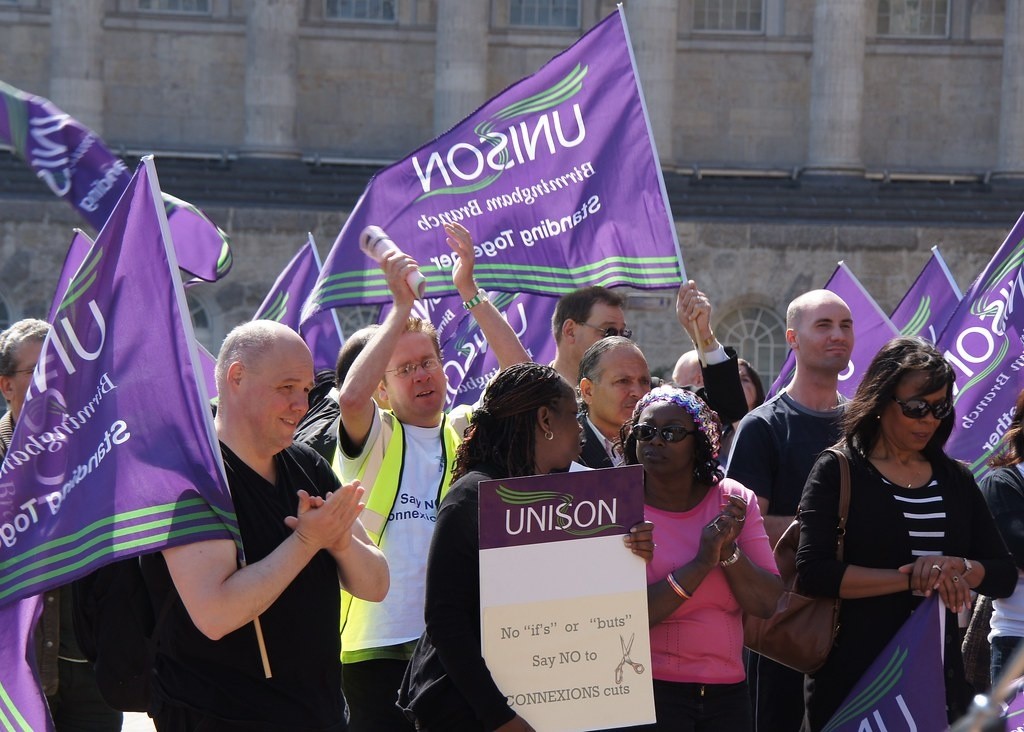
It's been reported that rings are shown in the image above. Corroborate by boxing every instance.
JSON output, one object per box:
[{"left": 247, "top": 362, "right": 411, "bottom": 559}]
[
  {"left": 651, "top": 543, "right": 657, "bottom": 552},
  {"left": 735, "top": 515, "right": 745, "bottom": 522},
  {"left": 714, "top": 523, "right": 721, "bottom": 532},
  {"left": 952, "top": 576, "right": 958, "bottom": 582},
  {"left": 931, "top": 565, "right": 942, "bottom": 571}
]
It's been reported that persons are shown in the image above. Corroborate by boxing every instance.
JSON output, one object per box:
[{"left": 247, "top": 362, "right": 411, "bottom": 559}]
[
  {"left": 551, "top": 277, "right": 857, "bottom": 732},
  {"left": 610, "top": 387, "right": 782, "bottom": 732},
  {"left": 794, "top": 339, "right": 1024, "bottom": 732},
  {"left": 0, "top": 320, "right": 74, "bottom": 732},
  {"left": 397, "top": 361, "right": 655, "bottom": 732},
  {"left": 143, "top": 321, "right": 390, "bottom": 732},
  {"left": 330, "top": 223, "right": 533, "bottom": 732}
]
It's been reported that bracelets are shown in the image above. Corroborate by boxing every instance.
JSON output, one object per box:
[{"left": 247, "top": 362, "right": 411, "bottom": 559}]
[
  {"left": 720, "top": 545, "right": 740, "bottom": 567},
  {"left": 666, "top": 571, "right": 692, "bottom": 600},
  {"left": 692, "top": 330, "right": 715, "bottom": 349}
]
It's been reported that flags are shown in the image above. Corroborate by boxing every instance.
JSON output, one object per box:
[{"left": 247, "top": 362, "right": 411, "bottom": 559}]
[
  {"left": 252, "top": 9, "right": 683, "bottom": 413},
  {"left": 765, "top": 212, "right": 1024, "bottom": 484},
  {"left": 821, "top": 591, "right": 947, "bottom": 732},
  {"left": 0, "top": 84, "right": 244, "bottom": 732}
]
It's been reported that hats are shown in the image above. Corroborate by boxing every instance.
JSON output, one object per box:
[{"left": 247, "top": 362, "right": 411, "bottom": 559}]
[{"left": 633, "top": 385, "right": 721, "bottom": 459}]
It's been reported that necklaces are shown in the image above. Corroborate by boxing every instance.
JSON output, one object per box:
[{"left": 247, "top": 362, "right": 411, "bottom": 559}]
[{"left": 878, "top": 455, "right": 923, "bottom": 487}]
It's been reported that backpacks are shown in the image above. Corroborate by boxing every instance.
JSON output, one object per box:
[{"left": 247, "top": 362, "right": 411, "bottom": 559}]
[{"left": 72, "top": 558, "right": 178, "bottom": 712}]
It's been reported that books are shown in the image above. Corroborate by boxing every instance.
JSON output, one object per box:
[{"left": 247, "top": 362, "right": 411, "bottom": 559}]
[{"left": 359, "top": 226, "right": 426, "bottom": 301}]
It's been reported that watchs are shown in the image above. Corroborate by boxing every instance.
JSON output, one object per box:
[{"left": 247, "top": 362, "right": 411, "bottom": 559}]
[
  {"left": 961, "top": 558, "right": 971, "bottom": 579},
  {"left": 463, "top": 288, "right": 488, "bottom": 309}
]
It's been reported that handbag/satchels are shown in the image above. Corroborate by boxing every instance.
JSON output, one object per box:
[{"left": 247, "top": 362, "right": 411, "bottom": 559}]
[{"left": 742, "top": 448, "right": 851, "bottom": 672}]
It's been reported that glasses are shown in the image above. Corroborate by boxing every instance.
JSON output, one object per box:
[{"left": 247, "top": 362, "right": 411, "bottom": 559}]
[
  {"left": 633, "top": 425, "right": 696, "bottom": 443},
  {"left": 578, "top": 321, "right": 632, "bottom": 338},
  {"left": 892, "top": 397, "right": 953, "bottom": 419},
  {"left": 385, "top": 357, "right": 444, "bottom": 378}
]
